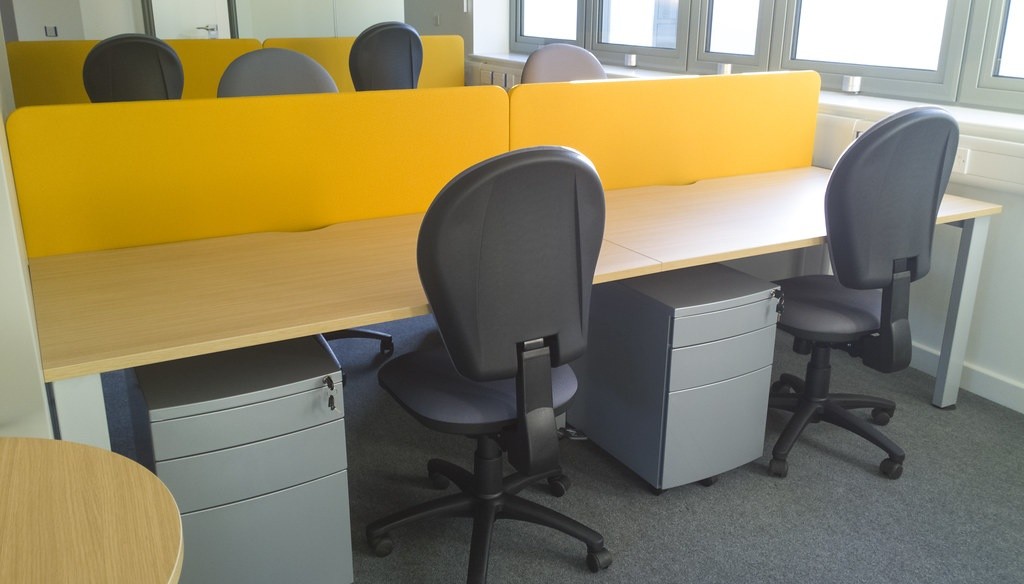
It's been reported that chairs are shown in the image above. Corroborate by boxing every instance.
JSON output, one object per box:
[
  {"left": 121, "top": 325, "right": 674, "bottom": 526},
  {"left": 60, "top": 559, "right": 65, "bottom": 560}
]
[
  {"left": 347, "top": 22, "right": 423, "bottom": 92},
  {"left": 769, "top": 106, "right": 960, "bottom": 481},
  {"left": 216, "top": 46, "right": 394, "bottom": 382},
  {"left": 82, "top": 33, "right": 184, "bottom": 104},
  {"left": 521, "top": 43, "right": 608, "bottom": 84},
  {"left": 365, "top": 146, "right": 613, "bottom": 584}
]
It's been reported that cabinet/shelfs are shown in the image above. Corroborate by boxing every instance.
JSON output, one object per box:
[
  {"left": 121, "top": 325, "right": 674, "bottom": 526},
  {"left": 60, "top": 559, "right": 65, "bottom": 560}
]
[
  {"left": 134, "top": 332, "right": 353, "bottom": 584},
  {"left": 565, "top": 261, "right": 781, "bottom": 496}
]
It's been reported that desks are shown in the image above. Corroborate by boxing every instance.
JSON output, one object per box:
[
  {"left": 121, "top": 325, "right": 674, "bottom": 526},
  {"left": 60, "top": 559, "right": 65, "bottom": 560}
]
[
  {"left": 0, "top": 435, "right": 183, "bottom": 584},
  {"left": 30, "top": 164, "right": 1005, "bottom": 451}
]
